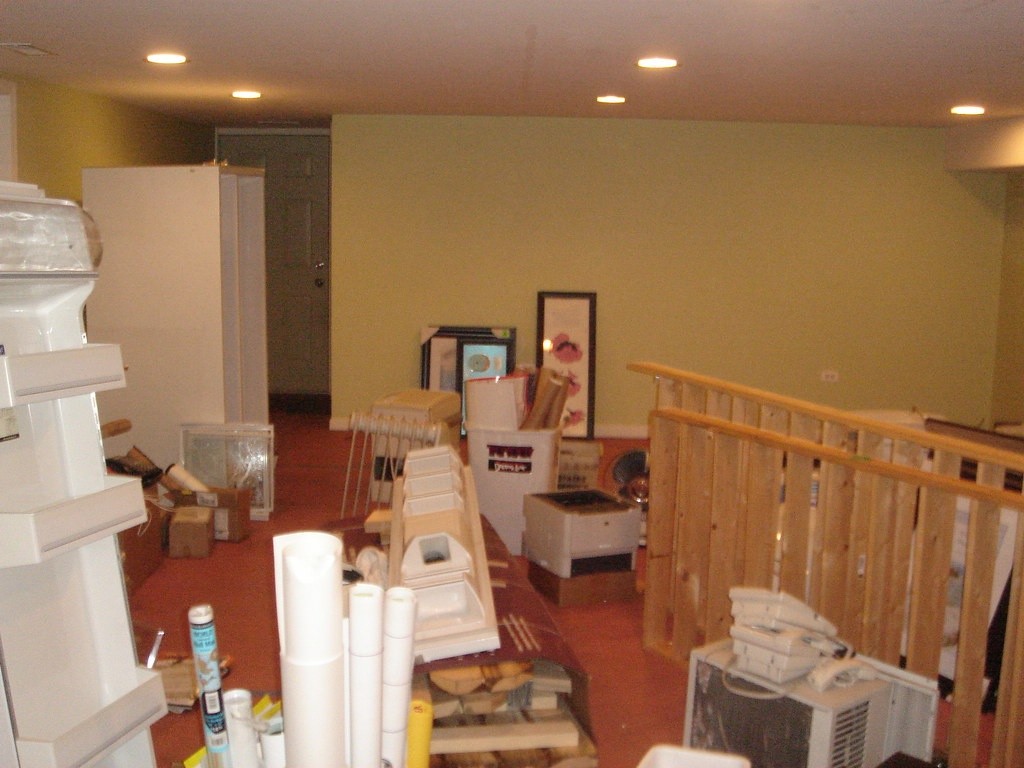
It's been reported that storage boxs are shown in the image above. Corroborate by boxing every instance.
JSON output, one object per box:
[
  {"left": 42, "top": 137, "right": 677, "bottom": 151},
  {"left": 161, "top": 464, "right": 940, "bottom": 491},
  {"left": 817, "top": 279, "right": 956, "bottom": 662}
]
[
  {"left": 389, "top": 514, "right": 595, "bottom": 768},
  {"left": 115, "top": 483, "right": 253, "bottom": 595}
]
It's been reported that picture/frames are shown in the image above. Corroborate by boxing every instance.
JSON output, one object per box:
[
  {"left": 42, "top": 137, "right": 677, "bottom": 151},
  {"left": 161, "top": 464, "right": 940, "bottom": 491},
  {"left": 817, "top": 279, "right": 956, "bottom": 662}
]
[
  {"left": 420, "top": 326, "right": 517, "bottom": 440},
  {"left": 536, "top": 290, "right": 595, "bottom": 442}
]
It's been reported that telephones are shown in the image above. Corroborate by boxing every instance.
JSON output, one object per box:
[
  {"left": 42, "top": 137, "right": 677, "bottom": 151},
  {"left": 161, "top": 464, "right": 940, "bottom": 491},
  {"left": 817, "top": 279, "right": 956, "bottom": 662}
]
[
  {"left": 729, "top": 586, "right": 840, "bottom": 636},
  {"left": 729, "top": 625, "right": 877, "bottom": 694}
]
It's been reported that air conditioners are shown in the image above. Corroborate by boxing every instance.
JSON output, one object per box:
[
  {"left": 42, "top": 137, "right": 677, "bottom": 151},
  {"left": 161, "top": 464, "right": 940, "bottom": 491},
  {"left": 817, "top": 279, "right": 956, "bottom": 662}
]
[{"left": 683, "top": 638, "right": 893, "bottom": 768}]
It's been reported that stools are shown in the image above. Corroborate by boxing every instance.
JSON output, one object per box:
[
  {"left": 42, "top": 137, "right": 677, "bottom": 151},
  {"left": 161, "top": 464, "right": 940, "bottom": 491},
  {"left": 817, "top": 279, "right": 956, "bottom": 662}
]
[{"left": 518, "top": 487, "right": 643, "bottom": 608}]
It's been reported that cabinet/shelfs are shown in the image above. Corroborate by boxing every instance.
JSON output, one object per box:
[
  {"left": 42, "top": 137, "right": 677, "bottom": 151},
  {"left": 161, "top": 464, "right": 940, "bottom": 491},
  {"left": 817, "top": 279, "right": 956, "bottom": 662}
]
[
  {"left": 0, "top": 184, "right": 170, "bottom": 768},
  {"left": 79, "top": 163, "right": 266, "bottom": 478},
  {"left": 369, "top": 389, "right": 459, "bottom": 503}
]
